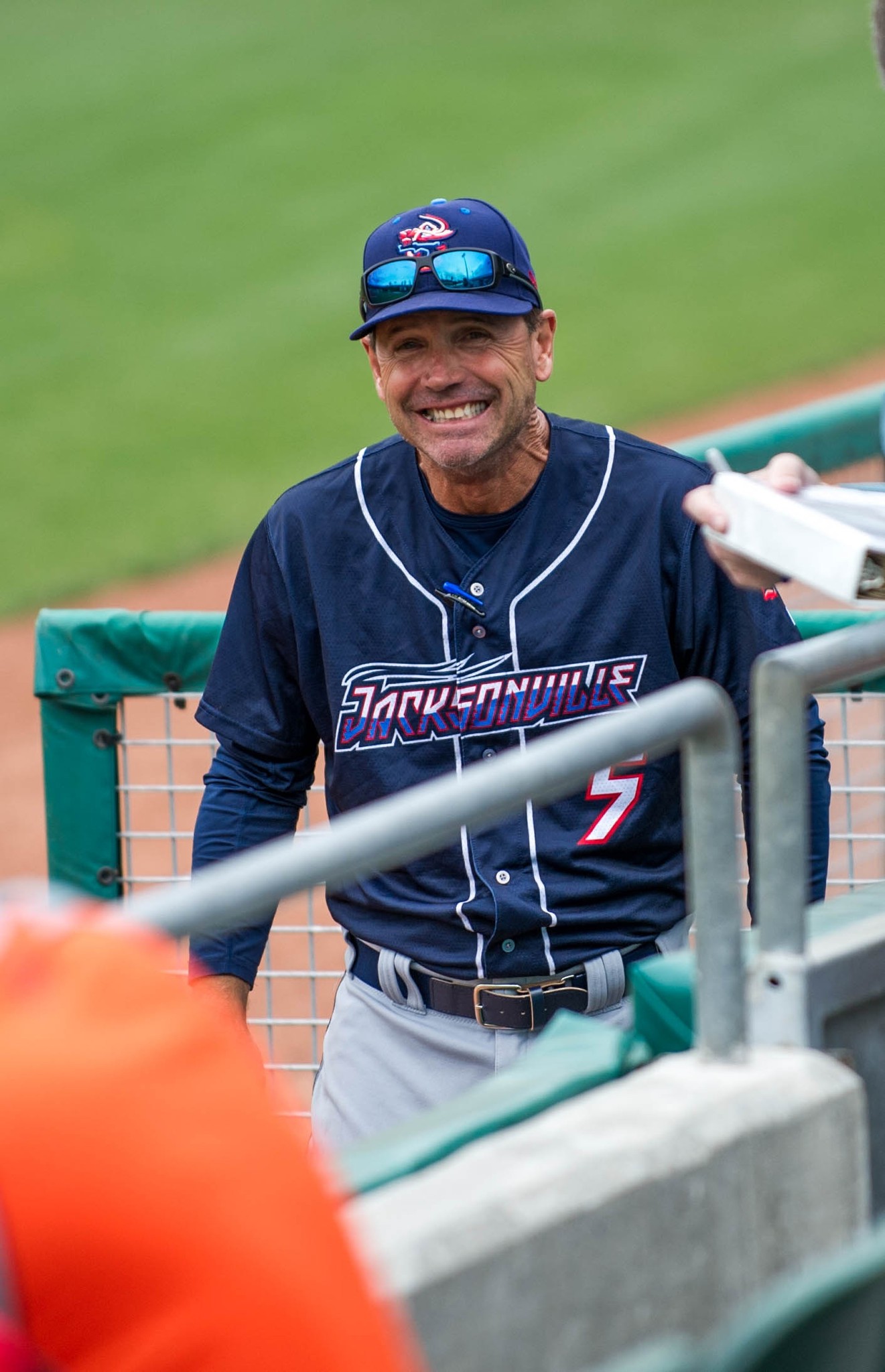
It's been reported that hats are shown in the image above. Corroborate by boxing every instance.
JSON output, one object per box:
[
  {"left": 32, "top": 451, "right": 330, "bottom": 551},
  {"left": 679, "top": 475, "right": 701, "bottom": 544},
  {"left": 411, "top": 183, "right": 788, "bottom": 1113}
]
[{"left": 349, "top": 196, "right": 541, "bottom": 342}]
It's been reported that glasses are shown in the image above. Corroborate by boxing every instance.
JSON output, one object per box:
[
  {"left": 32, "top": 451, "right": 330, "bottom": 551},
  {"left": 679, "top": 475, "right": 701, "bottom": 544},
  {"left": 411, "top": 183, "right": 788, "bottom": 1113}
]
[{"left": 359, "top": 248, "right": 543, "bottom": 323}]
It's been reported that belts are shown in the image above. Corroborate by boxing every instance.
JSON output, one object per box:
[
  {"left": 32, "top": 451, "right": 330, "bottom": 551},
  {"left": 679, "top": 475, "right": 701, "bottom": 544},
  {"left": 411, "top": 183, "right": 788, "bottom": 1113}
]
[{"left": 350, "top": 938, "right": 668, "bottom": 1034}]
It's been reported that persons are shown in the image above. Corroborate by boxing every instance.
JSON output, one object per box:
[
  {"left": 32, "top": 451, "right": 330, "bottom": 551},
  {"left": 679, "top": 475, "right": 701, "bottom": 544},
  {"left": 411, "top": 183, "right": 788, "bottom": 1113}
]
[
  {"left": 684, "top": 448, "right": 885, "bottom": 592},
  {"left": 186, "top": 188, "right": 831, "bottom": 1146}
]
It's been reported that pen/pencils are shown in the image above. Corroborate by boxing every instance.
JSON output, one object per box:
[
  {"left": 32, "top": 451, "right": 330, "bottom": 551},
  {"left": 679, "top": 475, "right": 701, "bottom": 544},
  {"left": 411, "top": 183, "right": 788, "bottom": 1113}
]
[{"left": 704, "top": 447, "right": 732, "bottom": 474}]
